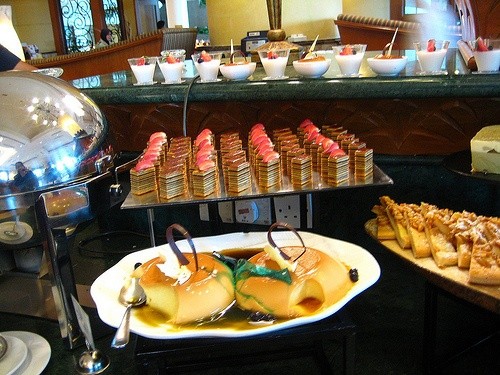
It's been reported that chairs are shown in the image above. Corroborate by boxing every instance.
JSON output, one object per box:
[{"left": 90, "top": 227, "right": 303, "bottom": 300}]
[{"left": 333, "top": 12, "right": 461, "bottom": 49}]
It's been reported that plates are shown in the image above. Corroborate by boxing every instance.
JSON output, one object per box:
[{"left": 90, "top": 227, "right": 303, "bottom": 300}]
[
  {"left": 30, "top": 67, "right": 63, "bottom": 78},
  {"left": 89, "top": 231, "right": 381, "bottom": 339},
  {"left": 0, "top": 331, "right": 52, "bottom": 375},
  {"left": 441, "top": 150, "right": 500, "bottom": 182},
  {"left": 0, "top": 221, "right": 34, "bottom": 245}
]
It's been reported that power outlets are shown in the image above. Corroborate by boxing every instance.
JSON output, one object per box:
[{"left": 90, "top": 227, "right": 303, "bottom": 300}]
[{"left": 273, "top": 194, "right": 312, "bottom": 229}]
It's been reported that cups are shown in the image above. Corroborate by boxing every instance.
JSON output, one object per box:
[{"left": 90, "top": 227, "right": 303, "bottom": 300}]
[
  {"left": 332, "top": 44, "right": 367, "bottom": 76},
  {"left": 128, "top": 57, "right": 157, "bottom": 83},
  {"left": 156, "top": 55, "right": 185, "bottom": 83},
  {"left": 413, "top": 41, "right": 450, "bottom": 72},
  {"left": 256, "top": 48, "right": 291, "bottom": 79},
  {"left": 191, "top": 50, "right": 223, "bottom": 81},
  {"left": 468, "top": 40, "right": 500, "bottom": 72}
]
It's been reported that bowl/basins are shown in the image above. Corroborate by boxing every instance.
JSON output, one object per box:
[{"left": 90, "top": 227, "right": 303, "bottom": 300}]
[
  {"left": 219, "top": 62, "right": 256, "bottom": 80},
  {"left": 367, "top": 55, "right": 409, "bottom": 77},
  {"left": 292, "top": 59, "right": 331, "bottom": 78}
]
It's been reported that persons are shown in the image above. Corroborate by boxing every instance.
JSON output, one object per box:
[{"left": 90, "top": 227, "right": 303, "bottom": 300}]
[
  {"left": 12, "top": 161, "right": 39, "bottom": 191},
  {"left": 42, "top": 162, "right": 59, "bottom": 183},
  {"left": 95, "top": 28, "right": 115, "bottom": 50}
]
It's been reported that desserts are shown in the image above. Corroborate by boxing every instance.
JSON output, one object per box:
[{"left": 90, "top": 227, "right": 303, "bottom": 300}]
[
  {"left": 125, "top": 222, "right": 353, "bottom": 325},
  {"left": 129, "top": 120, "right": 373, "bottom": 200}
]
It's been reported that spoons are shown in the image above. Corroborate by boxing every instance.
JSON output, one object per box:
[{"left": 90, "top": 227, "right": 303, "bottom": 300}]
[
  {"left": 0, "top": 335, "right": 8, "bottom": 360},
  {"left": 110, "top": 281, "right": 146, "bottom": 349},
  {"left": 3, "top": 215, "right": 20, "bottom": 237}
]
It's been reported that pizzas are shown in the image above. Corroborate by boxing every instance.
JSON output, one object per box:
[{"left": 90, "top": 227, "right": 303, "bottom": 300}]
[{"left": 371, "top": 194, "right": 500, "bottom": 287}]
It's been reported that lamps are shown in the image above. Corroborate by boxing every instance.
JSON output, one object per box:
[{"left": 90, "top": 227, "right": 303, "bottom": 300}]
[{"left": 0, "top": 10, "right": 27, "bottom": 62}]
